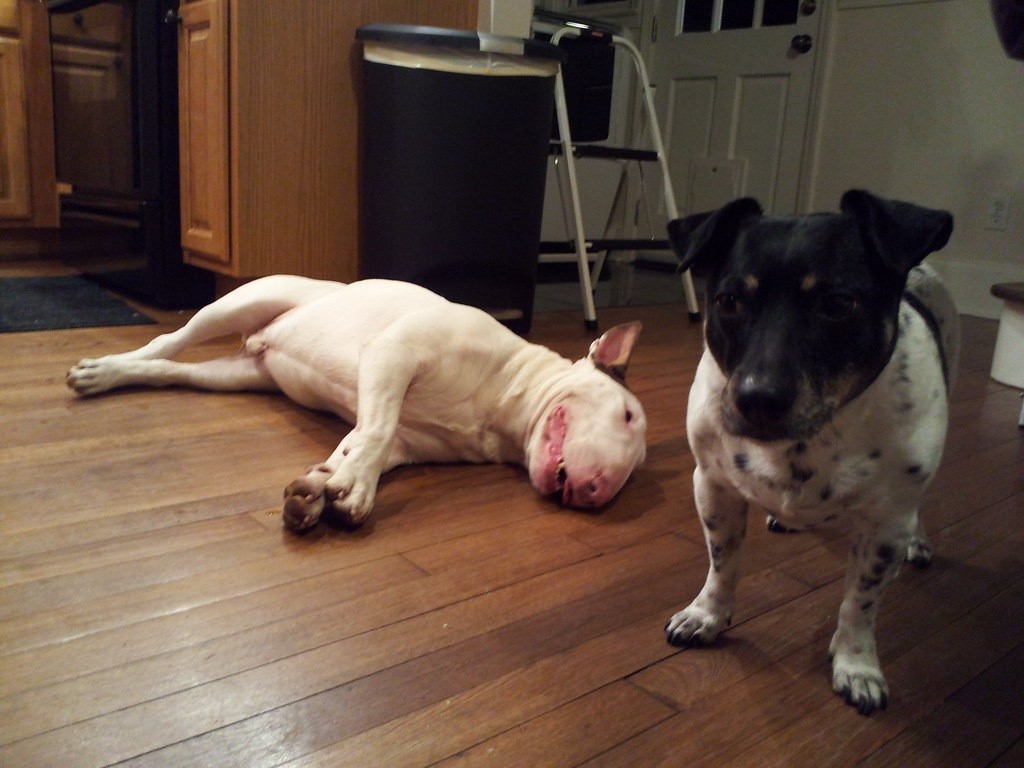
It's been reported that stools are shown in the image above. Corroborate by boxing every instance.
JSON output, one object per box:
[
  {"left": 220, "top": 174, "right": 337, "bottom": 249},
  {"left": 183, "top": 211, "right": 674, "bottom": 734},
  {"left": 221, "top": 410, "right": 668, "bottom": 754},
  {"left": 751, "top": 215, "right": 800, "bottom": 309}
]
[{"left": 988, "top": 281, "right": 1024, "bottom": 390}]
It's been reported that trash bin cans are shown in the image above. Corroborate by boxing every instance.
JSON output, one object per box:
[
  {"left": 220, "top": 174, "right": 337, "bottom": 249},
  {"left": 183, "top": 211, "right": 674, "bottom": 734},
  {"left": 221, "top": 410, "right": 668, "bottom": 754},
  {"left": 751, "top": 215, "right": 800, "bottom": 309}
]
[{"left": 355, "top": 23, "right": 565, "bottom": 334}]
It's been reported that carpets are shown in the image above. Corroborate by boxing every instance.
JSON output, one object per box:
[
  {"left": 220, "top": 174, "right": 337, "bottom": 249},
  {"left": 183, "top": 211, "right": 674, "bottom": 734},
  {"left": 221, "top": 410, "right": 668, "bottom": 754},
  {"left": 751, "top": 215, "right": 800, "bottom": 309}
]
[{"left": 0, "top": 274, "right": 160, "bottom": 333}]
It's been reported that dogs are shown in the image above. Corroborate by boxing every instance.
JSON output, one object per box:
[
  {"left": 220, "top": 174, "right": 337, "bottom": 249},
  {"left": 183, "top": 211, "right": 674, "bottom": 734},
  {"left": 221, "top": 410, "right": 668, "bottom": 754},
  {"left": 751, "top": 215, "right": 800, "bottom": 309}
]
[
  {"left": 663, "top": 186, "right": 964, "bottom": 720},
  {"left": 65, "top": 274, "right": 650, "bottom": 536}
]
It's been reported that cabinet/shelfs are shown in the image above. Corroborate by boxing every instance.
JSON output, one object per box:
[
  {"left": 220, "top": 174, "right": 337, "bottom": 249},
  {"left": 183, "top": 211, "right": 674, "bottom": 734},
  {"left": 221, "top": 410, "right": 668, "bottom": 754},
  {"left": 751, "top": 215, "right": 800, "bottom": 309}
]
[{"left": 0, "top": 0, "right": 480, "bottom": 301}]
